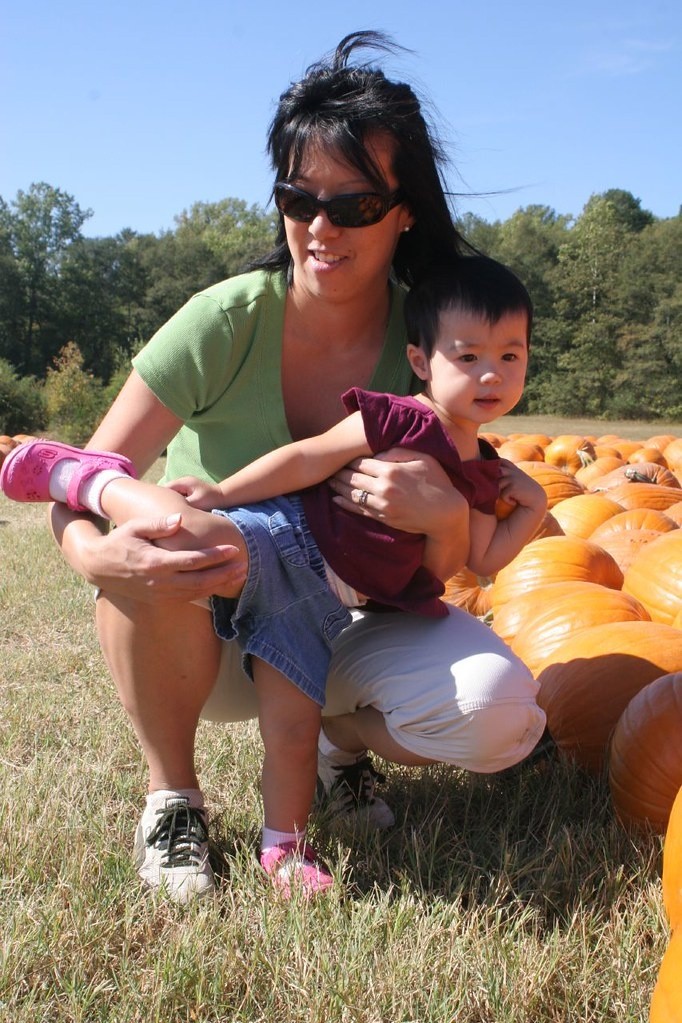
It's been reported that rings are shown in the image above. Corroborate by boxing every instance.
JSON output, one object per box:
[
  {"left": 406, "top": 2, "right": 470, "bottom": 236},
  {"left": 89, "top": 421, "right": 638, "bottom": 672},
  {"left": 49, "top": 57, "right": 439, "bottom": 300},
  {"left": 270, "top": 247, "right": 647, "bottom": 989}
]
[{"left": 358, "top": 490, "right": 374, "bottom": 505}]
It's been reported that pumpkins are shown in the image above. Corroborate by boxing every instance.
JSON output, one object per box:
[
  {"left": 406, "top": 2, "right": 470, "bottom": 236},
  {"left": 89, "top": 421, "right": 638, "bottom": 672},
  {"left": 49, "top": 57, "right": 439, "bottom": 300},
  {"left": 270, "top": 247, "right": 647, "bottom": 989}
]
[
  {"left": 442, "top": 432, "right": 682, "bottom": 1023},
  {"left": 0, "top": 434, "right": 34, "bottom": 471}
]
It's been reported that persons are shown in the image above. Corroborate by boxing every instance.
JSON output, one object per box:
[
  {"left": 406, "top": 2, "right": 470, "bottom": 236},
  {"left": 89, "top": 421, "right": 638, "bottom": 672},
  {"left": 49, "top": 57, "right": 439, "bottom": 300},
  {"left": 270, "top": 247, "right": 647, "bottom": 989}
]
[
  {"left": 1, "top": 257, "right": 547, "bottom": 908},
  {"left": 48, "top": 30, "right": 549, "bottom": 905}
]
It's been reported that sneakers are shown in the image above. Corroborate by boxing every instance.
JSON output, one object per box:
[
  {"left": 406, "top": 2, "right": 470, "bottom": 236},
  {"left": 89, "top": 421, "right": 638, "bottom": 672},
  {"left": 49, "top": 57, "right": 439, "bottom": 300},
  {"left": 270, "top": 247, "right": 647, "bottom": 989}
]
[
  {"left": 137, "top": 796, "right": 216, "bottom": 906},
  {"left": 317, "top": 749, "right": 395, "bottom": 834}
]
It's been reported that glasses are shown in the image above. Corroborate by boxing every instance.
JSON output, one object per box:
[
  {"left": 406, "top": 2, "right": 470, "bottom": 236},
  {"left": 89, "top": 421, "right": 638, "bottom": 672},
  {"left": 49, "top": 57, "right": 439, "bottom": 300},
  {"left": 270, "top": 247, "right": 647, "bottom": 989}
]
[{"left": 274, "top": 179, "right": 405, "bottom": 228}]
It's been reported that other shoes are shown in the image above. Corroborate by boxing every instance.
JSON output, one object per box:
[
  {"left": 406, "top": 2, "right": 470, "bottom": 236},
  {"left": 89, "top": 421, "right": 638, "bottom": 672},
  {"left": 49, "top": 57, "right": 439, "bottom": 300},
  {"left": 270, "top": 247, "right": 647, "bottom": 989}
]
[
  {"left": 260, "top": 841, "right": 347, "bottom": 909},
  {"left": 0, "top": 438, "right": 137, "bottom": 512}
]
